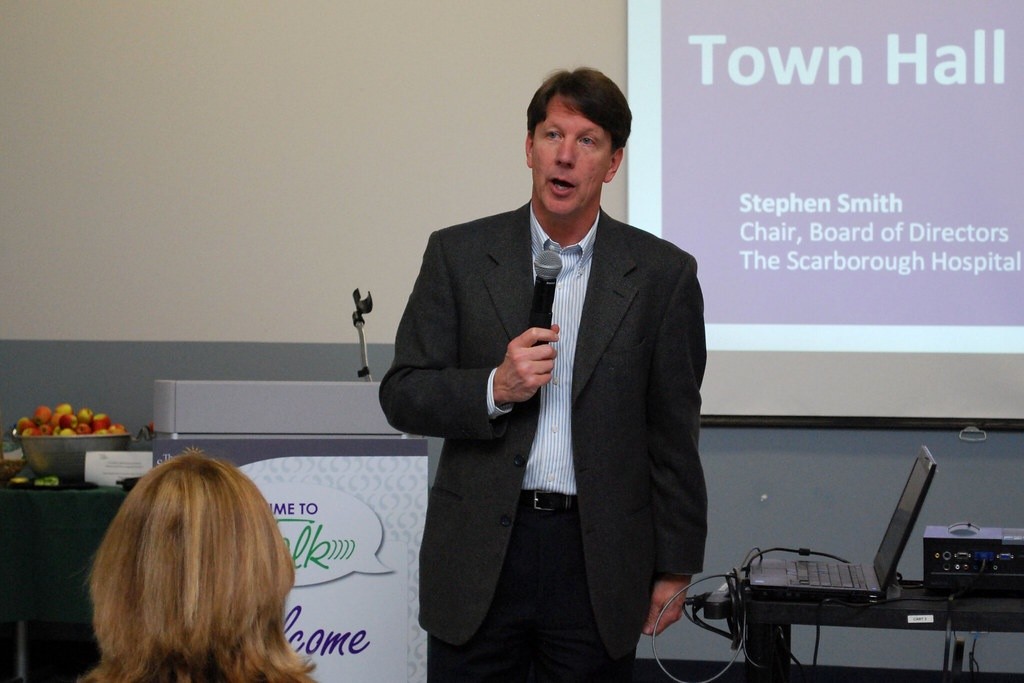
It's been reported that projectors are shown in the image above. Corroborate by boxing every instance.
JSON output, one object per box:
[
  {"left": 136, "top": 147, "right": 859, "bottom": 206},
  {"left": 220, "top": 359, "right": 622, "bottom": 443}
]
[{"left": 926, "top": 524, "right": 1024, "bottom": 599}]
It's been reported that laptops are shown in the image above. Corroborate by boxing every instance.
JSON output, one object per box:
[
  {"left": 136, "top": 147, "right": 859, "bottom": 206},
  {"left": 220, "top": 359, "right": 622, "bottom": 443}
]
[{"left": 749, "top": 445, "right": 937, "bottom": 601}]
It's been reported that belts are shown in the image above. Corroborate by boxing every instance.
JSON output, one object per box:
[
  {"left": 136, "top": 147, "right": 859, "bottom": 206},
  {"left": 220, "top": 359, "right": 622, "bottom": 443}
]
[{"left": 519, "top": 488, "right": 578, "bottom": 511}]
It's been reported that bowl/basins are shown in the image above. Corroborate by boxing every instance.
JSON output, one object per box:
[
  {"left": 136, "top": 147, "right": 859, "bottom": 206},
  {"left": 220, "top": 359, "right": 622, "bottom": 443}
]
[{"left": 15, "top": 434, "right": 133, "bottom": 480}]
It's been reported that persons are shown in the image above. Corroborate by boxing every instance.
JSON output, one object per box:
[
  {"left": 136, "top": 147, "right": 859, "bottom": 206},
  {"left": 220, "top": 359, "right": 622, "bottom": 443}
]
[
  {"left": 379, "top": 68, "right": 707, "bottom": 683},
  {"left": 79, "top": 453, "right": 318, "bottom": 683}
]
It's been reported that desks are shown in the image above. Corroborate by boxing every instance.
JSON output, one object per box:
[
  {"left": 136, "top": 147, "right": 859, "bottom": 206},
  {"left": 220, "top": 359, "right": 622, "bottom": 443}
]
[
  {"left": 702, "top": 572, "right": 1024, "bottom": 682},
  {"left": 0, "top": 484, "right": 127, "bottom": 682}
]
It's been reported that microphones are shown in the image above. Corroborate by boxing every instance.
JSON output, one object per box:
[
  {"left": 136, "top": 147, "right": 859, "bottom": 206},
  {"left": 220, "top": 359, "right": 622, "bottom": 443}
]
[{"left": 528, "top": 249, "right": 561, "bottom": 347}]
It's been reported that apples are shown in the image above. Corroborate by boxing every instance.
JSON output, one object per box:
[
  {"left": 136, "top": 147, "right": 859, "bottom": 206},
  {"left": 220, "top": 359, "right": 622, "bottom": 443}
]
[{"left": 15, "top": 404, "right": 127, "bottom": 437}]
